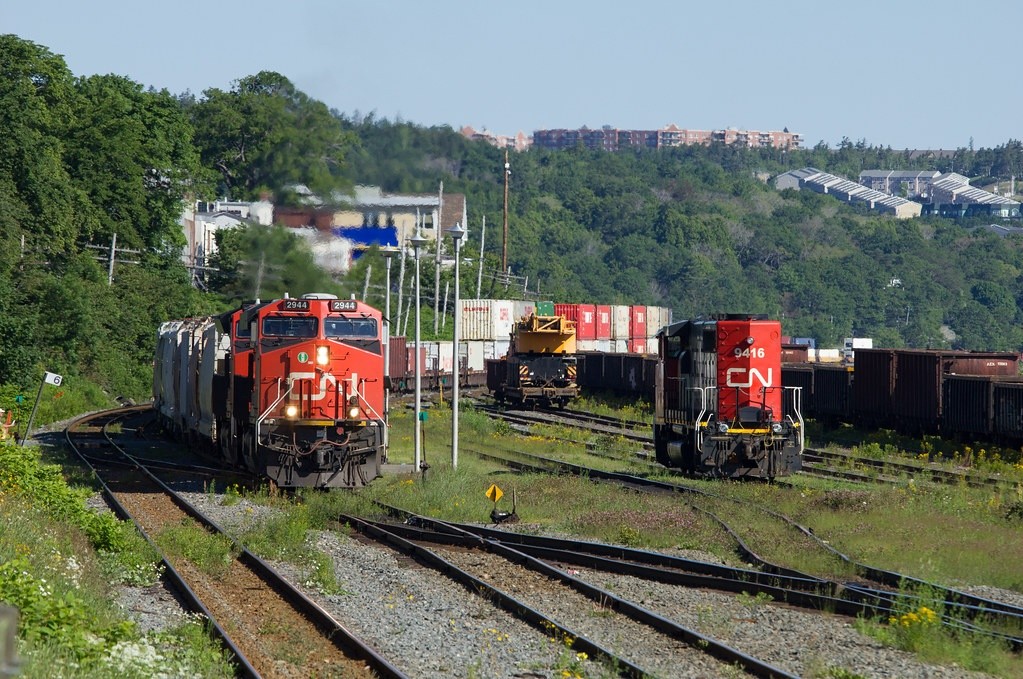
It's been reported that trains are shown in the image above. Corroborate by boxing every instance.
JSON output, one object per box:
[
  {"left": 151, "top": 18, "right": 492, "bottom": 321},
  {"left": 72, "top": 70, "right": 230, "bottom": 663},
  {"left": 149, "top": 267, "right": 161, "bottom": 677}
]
[
  {"left": 651, "top": 310, "right": 805, "bottom": 480},
  {"left": 154, "top": 296, "right": 674, "bottom": 488},
  {"left": 484, "top": 312, "right": 580, "bottom": 411}
]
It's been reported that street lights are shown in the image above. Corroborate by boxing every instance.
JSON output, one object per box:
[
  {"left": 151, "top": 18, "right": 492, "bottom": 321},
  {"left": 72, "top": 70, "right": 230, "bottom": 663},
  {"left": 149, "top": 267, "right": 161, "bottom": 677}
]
[
  {"left": 443, "top": 222, "right": 468, "bottom": 467},
  {"left": 407, "top": 230, "right": 432, "bottom": 472},
  {"left": 381, "top": 245, "right": 402, "bottom": 320}
]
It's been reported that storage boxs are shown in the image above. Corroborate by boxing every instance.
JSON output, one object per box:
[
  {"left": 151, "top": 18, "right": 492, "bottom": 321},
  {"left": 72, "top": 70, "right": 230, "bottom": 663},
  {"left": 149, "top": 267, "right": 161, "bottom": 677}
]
[{"left": 390, "top": 296, "right": 673, "bottom": 379}]
